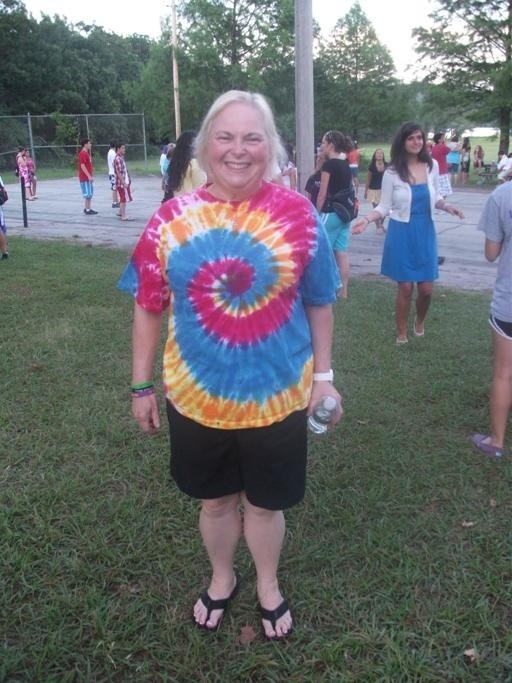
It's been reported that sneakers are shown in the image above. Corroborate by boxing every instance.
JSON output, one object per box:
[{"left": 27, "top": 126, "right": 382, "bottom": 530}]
[
  {"left": 414, "top": 316, "right": 424, "bottom": 336},
  {"left": 471, "top": 434, "right": 503, "bottom": 457},
  {"left": 112, "top": 204, "right": 119, "bottom": 208},
  {"left": 86, "top": 210, "right": 98, "bottom": 214},
  {"left": 396, "top": 336, "right": 408, "bottom": 344}
]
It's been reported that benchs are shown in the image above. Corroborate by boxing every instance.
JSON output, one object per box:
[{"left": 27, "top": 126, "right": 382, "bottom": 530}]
[{"left": 474, "top": 160, "right": 512, "bottom": 185}]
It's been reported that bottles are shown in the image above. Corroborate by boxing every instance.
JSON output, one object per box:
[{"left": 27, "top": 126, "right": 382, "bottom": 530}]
[{"left": 306, "top": 394, "right": 337, "bottom": 437}]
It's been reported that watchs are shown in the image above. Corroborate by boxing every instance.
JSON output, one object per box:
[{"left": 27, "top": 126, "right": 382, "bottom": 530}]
[{"left": 314, "top": 368, "right": 335, "bottom": 383}]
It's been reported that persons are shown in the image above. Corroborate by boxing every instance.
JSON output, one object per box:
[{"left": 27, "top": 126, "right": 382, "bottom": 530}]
[
  {"left": 128, "top": 90, "right": 344, "bottom": 638},
  {"left": 77, "top": 130, "right": 206, "bottom": 221},
  {"left": 13, "top": 146, "right": 39, "bottom": 199},
  {"left": 471, "top": 157, "right": 512, "bottom": 455},
  {"left": 0, "top": 176, "right": 10, "bottom": 257},
  {"left": 280, "top": 117, "right": 465, "bottom": 344},
  {"left": 466, "top": 135, "right": 512, "bottom": 181}
]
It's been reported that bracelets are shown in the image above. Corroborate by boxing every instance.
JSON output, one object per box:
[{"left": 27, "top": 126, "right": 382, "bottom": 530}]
[
  {"left": 130, "top": 385, "right": 153, "bottom": 392},
  {"left": 131, "top": 380, "right": 152, "bottom": 388},
  {"left": 131, "top": 387, "right": 155, "bottom": 397}
]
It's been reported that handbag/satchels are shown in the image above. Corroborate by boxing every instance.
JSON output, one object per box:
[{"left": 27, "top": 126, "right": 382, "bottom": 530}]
[{"left": 330, "top": 186, "right": 359, "bottom": 224}]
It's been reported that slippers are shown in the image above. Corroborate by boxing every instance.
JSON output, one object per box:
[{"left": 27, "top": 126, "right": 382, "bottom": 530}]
[
  {"left": 192, "top": 575, "right": 239, "bottom": 630},
  {"left": 258, "top": 598, "right": 294, "bottom": 639}
]
